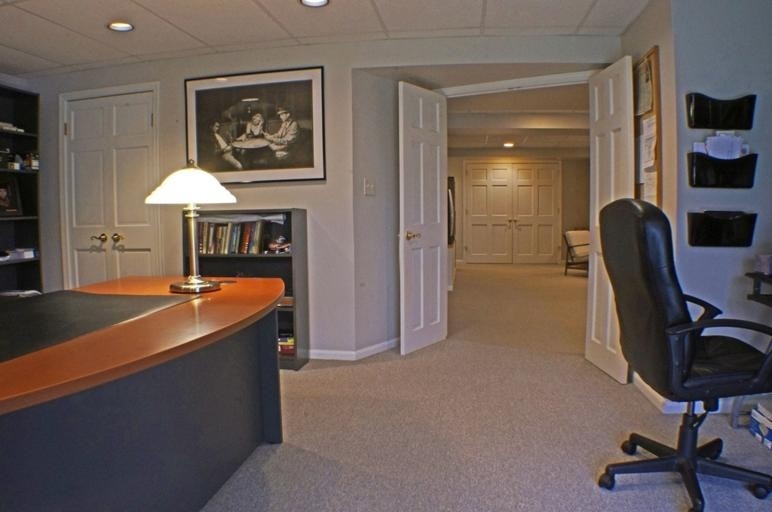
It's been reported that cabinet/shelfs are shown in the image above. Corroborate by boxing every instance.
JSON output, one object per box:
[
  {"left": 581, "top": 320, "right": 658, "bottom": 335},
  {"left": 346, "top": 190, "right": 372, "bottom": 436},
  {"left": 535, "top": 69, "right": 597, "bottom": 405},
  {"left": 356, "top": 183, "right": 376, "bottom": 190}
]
[
  {"left": 0, "top": 83, "right": 45, "bottom": 300},
  {"left": 182, "top": 206, "right": 310, "bottom": 371}
]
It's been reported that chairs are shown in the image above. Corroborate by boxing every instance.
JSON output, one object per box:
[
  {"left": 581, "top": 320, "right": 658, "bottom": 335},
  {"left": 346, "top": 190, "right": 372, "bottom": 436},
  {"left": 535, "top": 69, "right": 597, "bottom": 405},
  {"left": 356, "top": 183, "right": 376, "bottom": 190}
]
[
  {"left": 599, "top": 198, "right": 771, "bottom": 511},
  {"left": 563, "top": 229, "right": 590, "bottom": 276}
]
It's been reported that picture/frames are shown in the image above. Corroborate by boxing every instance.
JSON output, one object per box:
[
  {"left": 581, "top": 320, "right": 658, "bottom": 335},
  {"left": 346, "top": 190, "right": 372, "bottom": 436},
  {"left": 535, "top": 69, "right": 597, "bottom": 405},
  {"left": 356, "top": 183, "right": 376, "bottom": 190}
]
[{"left": 184, "top": 66, "right": 326, "bottom": 182}]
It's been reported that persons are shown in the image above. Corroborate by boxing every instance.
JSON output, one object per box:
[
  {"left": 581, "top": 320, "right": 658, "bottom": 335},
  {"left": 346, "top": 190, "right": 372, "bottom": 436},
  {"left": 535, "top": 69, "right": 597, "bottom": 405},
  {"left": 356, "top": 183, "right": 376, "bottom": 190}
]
[{"left": 208, "top": 106, "right": 301, "bottom": 170}]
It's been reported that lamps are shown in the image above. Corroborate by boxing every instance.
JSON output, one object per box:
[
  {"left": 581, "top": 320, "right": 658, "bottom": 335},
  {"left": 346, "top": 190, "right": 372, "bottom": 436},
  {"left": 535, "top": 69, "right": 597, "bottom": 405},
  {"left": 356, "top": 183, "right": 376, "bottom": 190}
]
[{"left": 145, "top": 165, "right": 239, "bottom": 294}]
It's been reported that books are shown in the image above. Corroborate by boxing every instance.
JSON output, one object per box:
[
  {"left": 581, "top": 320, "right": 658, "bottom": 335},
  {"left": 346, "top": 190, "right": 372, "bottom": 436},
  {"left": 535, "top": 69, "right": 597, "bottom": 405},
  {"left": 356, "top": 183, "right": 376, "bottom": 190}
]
[
  {"left": 198, "top": 222, "right": 262, "bottom": 255},
  {"left": 278, "top": 337, "right": 296, "bottom": 357},
  {"left": 749, "top": 399, "right": 772, "bottom": 451}
]
